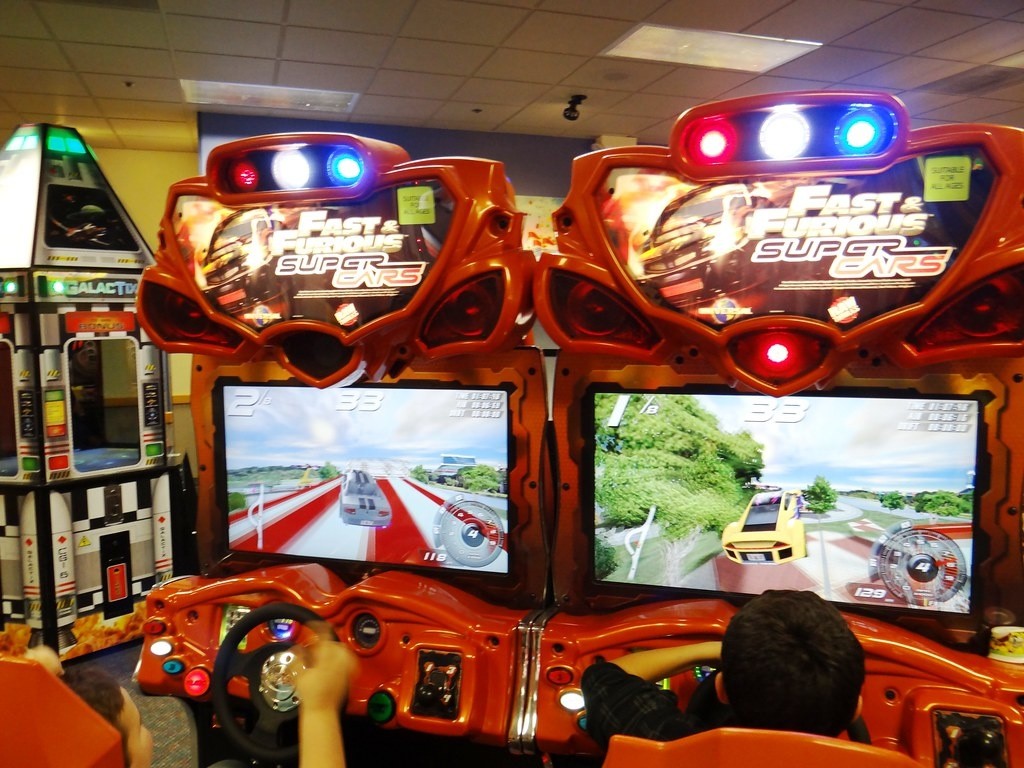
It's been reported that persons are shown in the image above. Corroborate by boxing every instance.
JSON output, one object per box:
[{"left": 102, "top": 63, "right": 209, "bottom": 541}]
[
  {"left": 580, "top": 589, "right": 865, "bottom": 754},
  {"left": 22, "top": 621, "right": 358, "bottom": 768}
]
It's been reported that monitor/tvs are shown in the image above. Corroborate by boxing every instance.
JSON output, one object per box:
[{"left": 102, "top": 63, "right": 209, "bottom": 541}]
[
  {"left": 581, "top": 381, "right": 992, "bottom": 616},
  {"left": 65, "top": 336, "right": 144, "bottom": 475},
  {"left": 211, "top": 374, "right": 520, "bottom": 588},
  {"left": 0, "top": 339, "right": 21, "bottom": 479}
]
